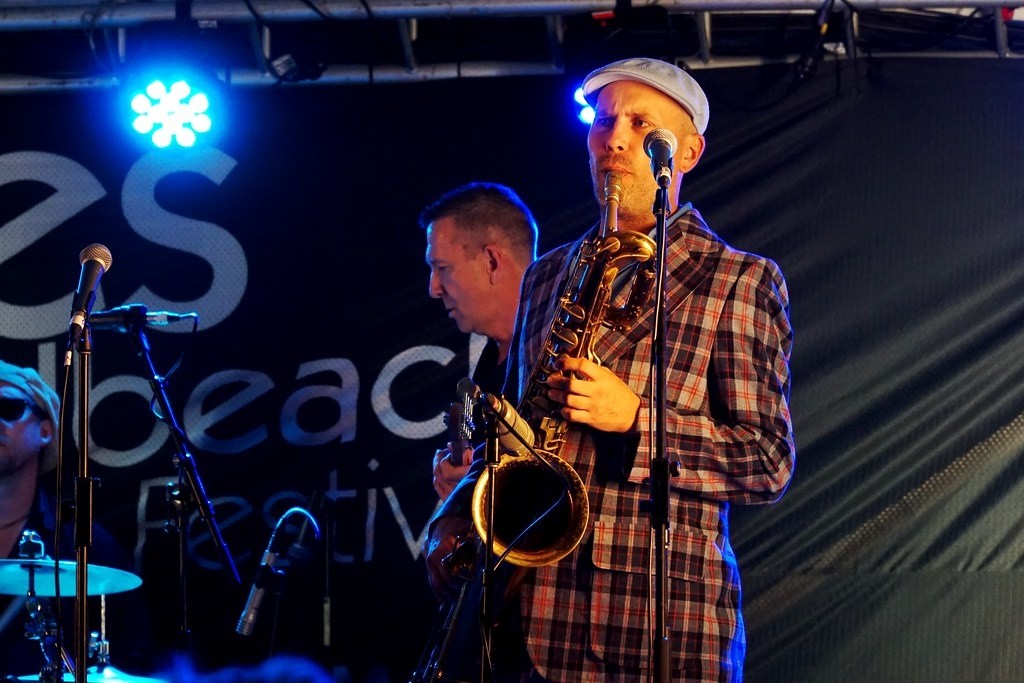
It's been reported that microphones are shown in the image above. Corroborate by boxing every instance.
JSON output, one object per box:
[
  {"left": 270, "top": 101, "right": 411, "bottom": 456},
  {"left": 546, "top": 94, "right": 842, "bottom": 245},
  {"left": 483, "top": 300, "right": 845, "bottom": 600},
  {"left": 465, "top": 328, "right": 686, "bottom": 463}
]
[
  {"left": 89, "top": 309, "right": 180, "bottom": 331},
  {"left": 794, "top": 0, "right": 835, "bottom": 80},
  {"left": 68, "top": 243, "right": 113, "bottom": 341},
  {"left": 235, "top": 527, "right": 282, "bottom": 635},
  {"left": 643, "top": 128, "right": 678, "bottom": 189},
  {"left": 477, "top": 391, "right": 534, "bottom": 457}
]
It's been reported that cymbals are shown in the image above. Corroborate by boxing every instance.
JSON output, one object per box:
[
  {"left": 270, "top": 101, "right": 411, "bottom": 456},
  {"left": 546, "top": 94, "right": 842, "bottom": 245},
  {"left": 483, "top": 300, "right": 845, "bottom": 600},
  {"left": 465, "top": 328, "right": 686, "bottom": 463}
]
[
  {"left": 1, "top": 557, "right": 144, "bottom": 597},
  {"left": 17, "top": 665, "right": 167, "bottom": 683}
]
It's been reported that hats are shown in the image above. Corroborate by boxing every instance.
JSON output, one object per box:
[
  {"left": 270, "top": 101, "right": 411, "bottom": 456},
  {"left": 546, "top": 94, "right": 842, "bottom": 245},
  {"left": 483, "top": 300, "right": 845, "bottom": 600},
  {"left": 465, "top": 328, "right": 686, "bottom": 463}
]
[
  {"left": 582, "top": 58, "right": 709, "bottom": 134},
  {"left": 0, "top": 358, "right": 61, "bottom": 467}
]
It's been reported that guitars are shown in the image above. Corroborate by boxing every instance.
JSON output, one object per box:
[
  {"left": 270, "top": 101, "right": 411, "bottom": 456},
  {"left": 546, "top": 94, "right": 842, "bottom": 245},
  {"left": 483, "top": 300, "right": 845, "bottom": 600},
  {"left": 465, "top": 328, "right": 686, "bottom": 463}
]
[{"left": 441, "top": 376, "right": 482, "bottom": 464}]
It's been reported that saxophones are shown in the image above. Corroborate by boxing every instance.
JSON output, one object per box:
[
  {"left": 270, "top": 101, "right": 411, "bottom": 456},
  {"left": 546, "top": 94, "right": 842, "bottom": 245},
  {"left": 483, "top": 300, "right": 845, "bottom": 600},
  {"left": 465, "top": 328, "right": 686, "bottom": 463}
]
[{"left": 471, "top": 168, "right": 657, "bottom": 571}]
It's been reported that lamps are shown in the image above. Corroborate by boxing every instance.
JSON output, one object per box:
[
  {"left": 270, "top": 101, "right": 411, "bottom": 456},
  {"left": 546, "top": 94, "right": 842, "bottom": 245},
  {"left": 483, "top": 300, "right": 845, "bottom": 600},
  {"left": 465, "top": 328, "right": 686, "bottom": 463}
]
[
  {"left": 570, "top": 74, "right": 596, "bottom": 128},
  {"left": 116, "top": 49, "right": 231, "bottom": 154}
]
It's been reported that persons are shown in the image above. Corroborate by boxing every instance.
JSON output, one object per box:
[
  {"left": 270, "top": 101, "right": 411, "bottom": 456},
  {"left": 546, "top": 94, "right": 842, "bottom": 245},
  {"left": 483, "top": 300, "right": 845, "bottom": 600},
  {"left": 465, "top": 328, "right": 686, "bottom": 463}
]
[
  {"left": 427, "top": 57, "right": 795, "bottom": 683},
  {"left": 420, "top": 184, "right": 539, "bottom": 503},
  {"left": 0, "top": 359, "right": 167, "bottom": 683}
]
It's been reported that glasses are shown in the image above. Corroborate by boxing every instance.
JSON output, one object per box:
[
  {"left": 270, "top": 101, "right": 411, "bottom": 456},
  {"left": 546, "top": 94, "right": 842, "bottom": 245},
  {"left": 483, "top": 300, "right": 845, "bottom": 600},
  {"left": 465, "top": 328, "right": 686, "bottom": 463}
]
[{"left": 0, "top": 396, "right": 52, "bottom": 421}]
